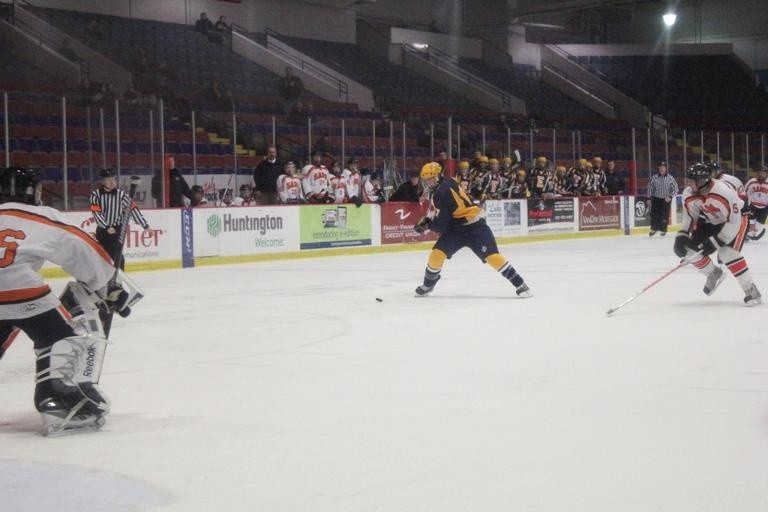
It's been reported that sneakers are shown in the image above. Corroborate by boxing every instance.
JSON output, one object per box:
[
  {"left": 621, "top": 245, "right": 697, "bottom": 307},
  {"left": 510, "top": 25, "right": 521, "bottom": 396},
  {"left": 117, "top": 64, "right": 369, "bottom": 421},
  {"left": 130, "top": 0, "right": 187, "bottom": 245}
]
[
  {"left": 742, "top": 283, "right": 762, "bottom": 302},
  {"left": 702, "top": 266, "right": 724, "bottom": 295},
  {"left": 649, "top": 229, "right": 666, "bottom": 236}
]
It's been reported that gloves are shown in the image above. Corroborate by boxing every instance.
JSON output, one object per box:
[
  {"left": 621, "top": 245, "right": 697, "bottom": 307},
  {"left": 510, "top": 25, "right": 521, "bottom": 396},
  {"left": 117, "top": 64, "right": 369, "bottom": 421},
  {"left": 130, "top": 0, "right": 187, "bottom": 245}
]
[
  {"left": 696, "top": 234, "right": 726, "bottom": 258},
  {"left": 413, "top": 216, "right": 436, "bottom": 234},
  {"left": 673, "top": 229, "right": 700, "bottom": 261}
]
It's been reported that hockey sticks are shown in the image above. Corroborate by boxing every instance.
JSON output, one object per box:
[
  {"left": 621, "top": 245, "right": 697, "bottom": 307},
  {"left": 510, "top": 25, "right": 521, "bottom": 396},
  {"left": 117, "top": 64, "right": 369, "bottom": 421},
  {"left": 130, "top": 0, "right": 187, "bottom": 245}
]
[
  {"left": 113, "top": 175, "right": 140, "bottom": 295},
  {"left": 606, "top": 249, "right": 704, "bottom": 313},
  {"left": 376, "top": 294, "right": 525, "bottom": 303}
]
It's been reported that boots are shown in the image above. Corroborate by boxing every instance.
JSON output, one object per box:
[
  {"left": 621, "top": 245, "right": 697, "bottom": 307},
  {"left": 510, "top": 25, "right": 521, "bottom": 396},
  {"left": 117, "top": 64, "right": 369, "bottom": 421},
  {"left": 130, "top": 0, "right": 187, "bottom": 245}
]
[
  {"left": 32, "top": 354, "right": 97, "bottom": 421},
  {"left": 509, "top": 274, "right": 530, "bottom": 295},
  {"left": 415, "top": 275, "right": 441, "bottom": 295}
]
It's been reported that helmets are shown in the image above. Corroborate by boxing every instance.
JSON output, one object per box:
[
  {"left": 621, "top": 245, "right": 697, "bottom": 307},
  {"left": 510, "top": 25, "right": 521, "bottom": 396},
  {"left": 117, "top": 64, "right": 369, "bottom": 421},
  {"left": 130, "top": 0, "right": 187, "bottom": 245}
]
[
  {"left": 704, "top": 160, "right": 721, "bottom": 171},
  {"left": 218, "top": 188, "right": 234, "bottom": 196},
  {"left": 191, "top": 183, "right": 204, "bottom": 193},
  {"left": 686, "top": 162, "right": 713, "bottom": 180},
  {"left": 0, "top": 166, "right": 45, "bottom": 206},
  {"left": 457, "top": 154, "right": 606, "bottom": 176},
  {"left": 420, "top": 160, "right": 443, "bottom": 180},
  {"left": 238, "top": 184, "right": 251, "bottom": 192}
]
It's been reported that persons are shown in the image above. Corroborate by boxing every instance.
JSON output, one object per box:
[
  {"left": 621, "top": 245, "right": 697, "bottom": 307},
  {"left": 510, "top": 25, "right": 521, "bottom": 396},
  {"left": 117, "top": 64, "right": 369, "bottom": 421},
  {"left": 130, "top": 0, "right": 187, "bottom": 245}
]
[
  {"left": 0, "top": 167, "right": 128, "bottom": 414},
  {"left": 199, "top": 12, "right": 233, "bottom": 40},
  {"left": 205, "top": 82, "right": 238, "bottom": 113},
  {"left": 438, "top": 151, "right": 626, "bottom": 199},
  {"left": 647, "top": 162, "right": 677, "bottom": 237},
  {"left": 150, "top": 120, "right": 430, "bottom": 209},
  {"left": 90, "top": 169, "right": 150, "bottom": 269},
  {"left": 673, "top": 161, "right": 767, "bottom": 304},
  {"left": 415, "top": 162, "right": 531, "bottom": 297},
  {"left": 278, "top": 68, "right": 319, "bottom": 127},
  {"left": 61, "top": 40, "right": 189, "bottom": 117}
]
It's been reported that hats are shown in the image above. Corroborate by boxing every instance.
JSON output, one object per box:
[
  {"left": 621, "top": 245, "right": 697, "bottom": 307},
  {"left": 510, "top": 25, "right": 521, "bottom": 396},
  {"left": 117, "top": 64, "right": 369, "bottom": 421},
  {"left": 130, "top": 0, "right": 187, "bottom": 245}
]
[
  {"left": 98, "top": 167, "right": 119, "bottom": 178},
  {"left": 656, "top": 159, "right": 668, "bottom": 167},
  {"left": 282, "top": 148, "right": 361, "bottom": 167}
]
